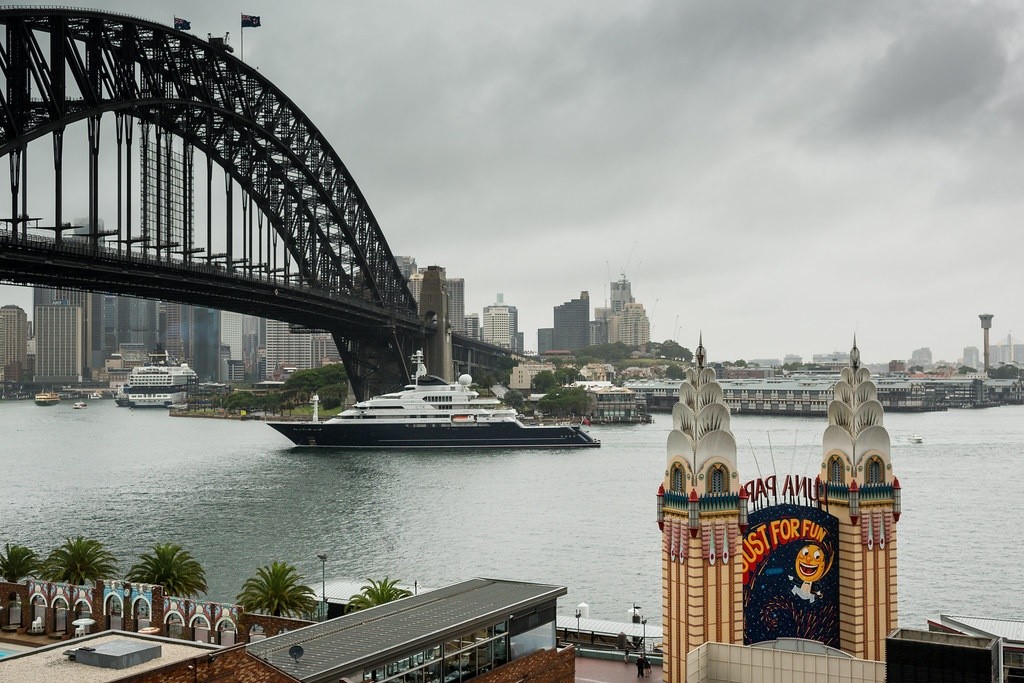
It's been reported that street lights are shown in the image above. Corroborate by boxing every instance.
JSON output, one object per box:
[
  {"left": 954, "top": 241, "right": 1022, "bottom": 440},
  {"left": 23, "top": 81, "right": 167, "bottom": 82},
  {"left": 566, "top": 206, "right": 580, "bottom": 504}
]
[
  {"left": 575, "top": 602, "right": 590, "bottom": 658},
  {"left": 317, "top": 552, "right": 329, "bottom": 622},
  {"left": 627, "top": 602, "right": 648, "bottom": 658}
]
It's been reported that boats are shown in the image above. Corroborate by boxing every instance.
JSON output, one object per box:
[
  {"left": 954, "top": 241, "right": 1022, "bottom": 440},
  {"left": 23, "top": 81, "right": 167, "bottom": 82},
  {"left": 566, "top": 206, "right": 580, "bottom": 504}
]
[
  {"left": 73, "top": 401, "right": 88, "bottom": 410},
  {"left": 266, "top": 347, "right": 601, "bottom": 449},
  {"left": 911, "top": 436, "right": 922, "bottom": 444},
  {"left": 34, "top": 392, "right": 60, "bottom": 406}
]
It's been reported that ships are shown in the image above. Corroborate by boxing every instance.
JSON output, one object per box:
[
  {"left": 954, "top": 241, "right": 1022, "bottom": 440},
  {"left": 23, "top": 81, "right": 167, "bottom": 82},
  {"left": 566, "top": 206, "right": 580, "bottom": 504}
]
[{"left": 114, "top": 342, "right": 199, "bottom": 407}]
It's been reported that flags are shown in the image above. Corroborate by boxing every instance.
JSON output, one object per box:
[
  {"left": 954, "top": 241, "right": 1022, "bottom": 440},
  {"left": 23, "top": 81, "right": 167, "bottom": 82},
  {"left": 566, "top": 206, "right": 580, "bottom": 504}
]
[
  {"left": 174, "top": 17, "right": 192, "bottom": 32},
  {"left": 584, "top": 417, "right": 591, "bottom": 426},
  {"left": 242, "top": 14, "right": 262, "bottom": 27}
]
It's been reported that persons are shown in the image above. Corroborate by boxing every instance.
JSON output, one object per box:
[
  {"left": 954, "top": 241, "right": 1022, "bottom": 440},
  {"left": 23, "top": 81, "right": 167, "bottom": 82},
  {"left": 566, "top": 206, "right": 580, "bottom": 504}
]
[{"left": 623, "top": 645, "right": 652, "bottom": 678}]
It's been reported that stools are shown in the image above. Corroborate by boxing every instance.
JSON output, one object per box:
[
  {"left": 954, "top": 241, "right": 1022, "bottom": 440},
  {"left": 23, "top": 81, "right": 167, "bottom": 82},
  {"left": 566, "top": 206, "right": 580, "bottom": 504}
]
[
  {"left": 75, "top": 625, "right": 85, "bottom": 638},
  {"left": 32, "top": 616, "right": 43, "bottom": 632}
]
